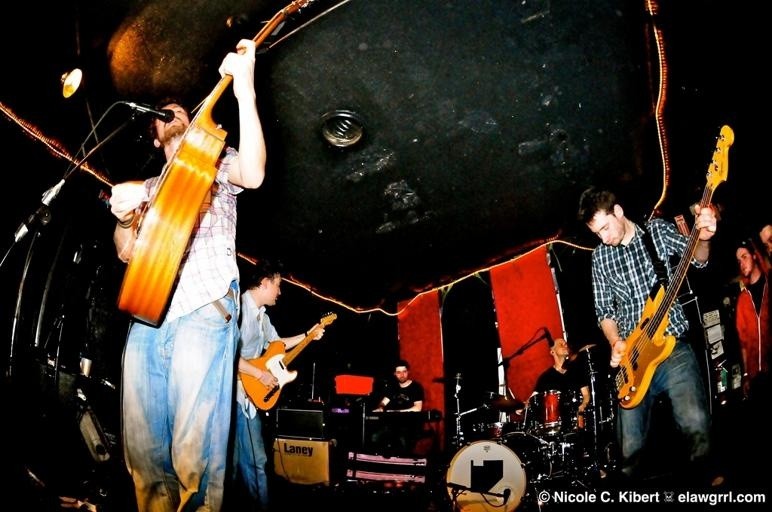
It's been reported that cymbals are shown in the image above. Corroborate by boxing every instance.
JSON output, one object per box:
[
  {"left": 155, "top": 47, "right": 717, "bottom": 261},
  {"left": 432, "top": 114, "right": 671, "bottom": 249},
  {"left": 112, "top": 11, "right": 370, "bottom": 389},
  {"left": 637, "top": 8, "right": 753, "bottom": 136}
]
[
  {"left": 578, "top": 343, "right": 596, "bottom": 352},
  {"left": 475, "top": 392, "right": 525, "bottom": 411}
]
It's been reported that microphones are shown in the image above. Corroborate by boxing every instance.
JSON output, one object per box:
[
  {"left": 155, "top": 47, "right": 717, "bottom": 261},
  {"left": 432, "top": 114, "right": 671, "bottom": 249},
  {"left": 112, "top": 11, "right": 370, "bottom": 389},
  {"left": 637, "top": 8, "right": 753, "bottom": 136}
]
[
  {"left": 454, "top": 372, "right": 462, "bottom": 397},
  {"left": 126, "top": 101, "right": 175, "bottom": 123},
  {"left": 504, "top": 488, "right": 510, "bottom": 504},
  {"left": 544, "top": 326, "right": 554, "bottom": 347}
]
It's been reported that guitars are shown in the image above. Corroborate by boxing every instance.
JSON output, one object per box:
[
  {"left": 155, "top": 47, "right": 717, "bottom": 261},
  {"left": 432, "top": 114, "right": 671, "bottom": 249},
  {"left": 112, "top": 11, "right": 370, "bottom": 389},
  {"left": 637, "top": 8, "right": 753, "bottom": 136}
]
[
  {"left": 240, "top": 311, "right": 338, "bottom": 410},
  {"left": 119, "top": 1, "right": 311, "bottom": 327},
  {"left": 611, "top": 124, "right": 729, "bottom": 410}
]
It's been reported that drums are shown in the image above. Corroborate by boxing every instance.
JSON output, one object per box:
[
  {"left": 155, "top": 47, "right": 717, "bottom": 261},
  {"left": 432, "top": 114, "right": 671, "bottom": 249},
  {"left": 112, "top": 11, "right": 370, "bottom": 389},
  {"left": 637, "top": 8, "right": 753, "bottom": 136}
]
[
  {"left": 528, "top": 389, "right": 578, "bottom": 436},
  {"left": 446, "top": 431, "right": 553, "bottom": 512},
  {"left": 488, "top": 422, "right": 515, "bottom": 441}
]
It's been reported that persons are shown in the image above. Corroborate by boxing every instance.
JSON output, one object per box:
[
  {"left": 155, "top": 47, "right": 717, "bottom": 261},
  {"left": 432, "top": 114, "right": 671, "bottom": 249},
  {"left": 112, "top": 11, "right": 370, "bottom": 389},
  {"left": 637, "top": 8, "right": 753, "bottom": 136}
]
[
  {"left": 725, "top": 237, "right": 772, "bottom": 400},
  {"left": 230, "top": 253, "right": 327, "bottom": 511},
  {"left": 530, "top": 336, "right": 593, "bottom": 413},
  {"left": 374, "top": 359, "right": 425, "bottom": 455},
  {"left": 573, "top": 182, "right": 727, "bottom": 488},
  {"left": 756, "top": 220, "right": 772, "bottom": 260},
  {"left": 107, "top": 38, "right": 270, "bottom": 512}
]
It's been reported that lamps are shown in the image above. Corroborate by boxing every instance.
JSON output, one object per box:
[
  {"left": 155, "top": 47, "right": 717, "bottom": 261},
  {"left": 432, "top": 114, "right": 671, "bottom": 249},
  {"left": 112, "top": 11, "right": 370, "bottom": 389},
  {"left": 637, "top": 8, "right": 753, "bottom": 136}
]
[{"left": 59, "top": 66, "right": 83, "bottom": 99}]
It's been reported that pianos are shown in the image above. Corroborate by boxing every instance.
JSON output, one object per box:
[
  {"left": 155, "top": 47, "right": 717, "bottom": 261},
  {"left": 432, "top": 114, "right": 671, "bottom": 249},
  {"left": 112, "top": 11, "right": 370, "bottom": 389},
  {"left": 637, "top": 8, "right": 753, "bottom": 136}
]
[{"left": 365, "top": 409, "right": 441, "bottom": 422}]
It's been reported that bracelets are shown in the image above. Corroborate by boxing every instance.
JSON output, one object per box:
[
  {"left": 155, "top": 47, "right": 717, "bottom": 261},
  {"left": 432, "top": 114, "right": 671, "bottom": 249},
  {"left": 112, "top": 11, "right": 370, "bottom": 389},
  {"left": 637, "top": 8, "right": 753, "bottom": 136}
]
[{"left": 304, "top": 330, "right": 308, "bottom": 338}]
